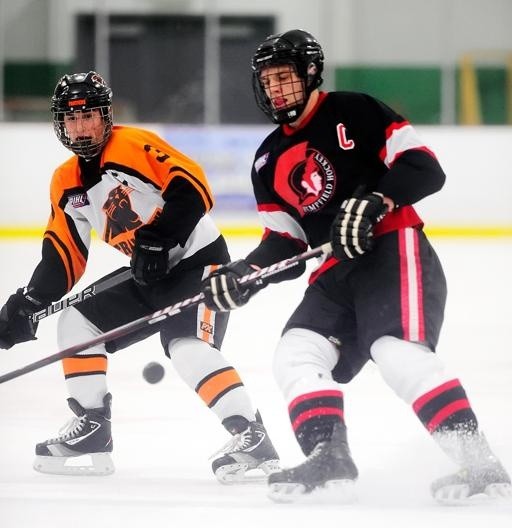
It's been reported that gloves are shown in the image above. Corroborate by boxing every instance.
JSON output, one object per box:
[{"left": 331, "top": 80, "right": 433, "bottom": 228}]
[
  {"left": 200, "top": 260, "right": 270, "bottom": 312},
  {"left": 129, "top": 224, "right": 171, "bottom": 288},
  {"left": 330, "top": 192, "right": 403, "bottom": 262},
  {"left": 0, "top": 286, "right": 52, "bottom": 351}
]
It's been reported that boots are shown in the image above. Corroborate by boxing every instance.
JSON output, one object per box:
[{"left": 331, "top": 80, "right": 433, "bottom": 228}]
[
  {"left": 431, "top": 429, "right": 512, "bottom": 500},
  {"left": 206, "top": 407, "right": 281, "bottom": 474},
  {"left": 268, "top": 420, "right": 360, "bottom": 487},
  {"left": 36, "top": 393, "right": 113, "bottom": 459}
]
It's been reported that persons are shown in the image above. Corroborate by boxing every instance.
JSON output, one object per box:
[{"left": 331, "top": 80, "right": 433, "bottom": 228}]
[
  {"left": 199, "top": 29, "right": 512, "bottom": 511},
  {"left": 0, "top": 71, "right": 285, "bottom": 485}
]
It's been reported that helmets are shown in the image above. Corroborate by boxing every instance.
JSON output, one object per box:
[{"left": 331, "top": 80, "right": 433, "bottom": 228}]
[
  {"left": 49, "top": 70, "right": 113, "bottom": 160},
  {"left": 250, "top": 29, "right": 325, "bottom": 124}
]
